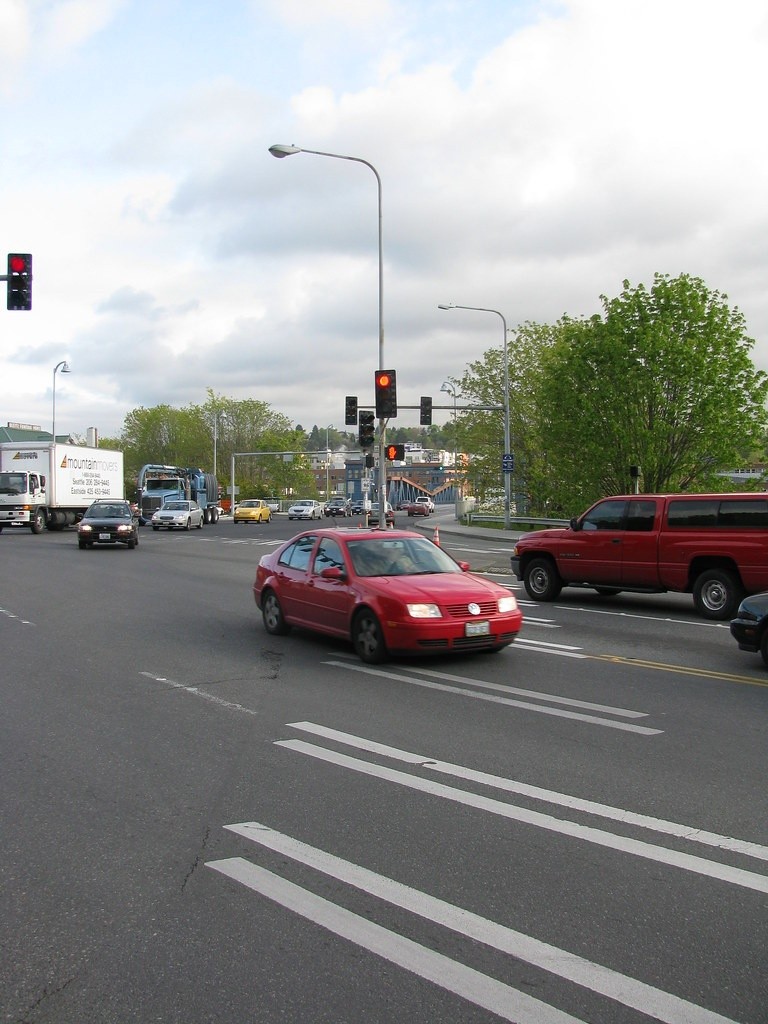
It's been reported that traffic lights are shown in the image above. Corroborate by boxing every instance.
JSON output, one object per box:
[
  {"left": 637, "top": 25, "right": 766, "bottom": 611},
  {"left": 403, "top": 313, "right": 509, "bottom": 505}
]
[
  {"left": 7, "top": 253, "right": 32, "bottom": 311},
  {"left": 387, "top": 445, "right": 404, "bottom": 460},
  {"left": 359, "top": 409, "right": 375, "bottom": 447},
  {"left": 375, "top": 370, "right": 397, "bottom": 419}
]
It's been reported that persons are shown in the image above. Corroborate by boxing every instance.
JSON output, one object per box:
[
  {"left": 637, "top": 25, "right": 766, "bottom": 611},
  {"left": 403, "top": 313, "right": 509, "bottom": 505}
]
[
  {"left": 382, "top": 548, "right": 412, "bottom": 572},
  {"left": 118, "top": 509, "right": 123, "bottom": 515},
  {"left": 183, "top": 505, "right": 187, "bottom": 509}
]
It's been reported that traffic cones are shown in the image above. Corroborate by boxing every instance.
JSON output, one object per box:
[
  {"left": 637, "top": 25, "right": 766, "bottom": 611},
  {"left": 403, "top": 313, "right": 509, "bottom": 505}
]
[
  {"left": 432, "top": 526, "right": 440, "bottom": 546},
  {"left": 389, "top": 522, "right": 394, "bottom": 529}
]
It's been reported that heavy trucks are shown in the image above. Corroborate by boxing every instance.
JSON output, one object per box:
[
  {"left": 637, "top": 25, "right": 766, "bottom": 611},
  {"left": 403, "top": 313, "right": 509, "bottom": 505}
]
[
  {"left": 0, "top": 441, "right": 124, "bottom": 535},
  {"left": 136, "top": 464, "right": 219, "bottom": 527}
]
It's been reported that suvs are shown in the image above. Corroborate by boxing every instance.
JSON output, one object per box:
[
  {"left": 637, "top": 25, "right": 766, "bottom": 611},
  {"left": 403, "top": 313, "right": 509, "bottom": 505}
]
[{"left": 415, "top": 497, "right": 435, "bottom": 513}]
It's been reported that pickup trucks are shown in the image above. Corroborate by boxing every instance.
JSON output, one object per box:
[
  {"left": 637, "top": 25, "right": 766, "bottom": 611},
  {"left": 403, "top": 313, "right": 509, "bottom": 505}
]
[{"left": 510, "top": 492, "right": 768, "bottom": 622}]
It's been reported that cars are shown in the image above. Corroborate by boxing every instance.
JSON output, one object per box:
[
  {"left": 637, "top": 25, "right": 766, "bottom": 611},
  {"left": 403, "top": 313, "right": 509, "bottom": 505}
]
[
  {"left": 351, "top": 500, "right": 364, "bottom": 515},
  {"left": 151, "top": 500, "right": 204, "bottom": 532},
  {"left": 408, "top": 502, "right": 429, "bottom": 517},
  {"left": 287, "top": 499, "right": 323, "bottom": 520},
  {"left": 76, "top": 498, "right": 140, "bottom": 549},
  {"left": 234, "top": 499, "right": 272, "bottom": 524},
  {"left": 325, "top": 500, "right": 353, "bottom": 517},
  {"left": 729, "top": 592, "right": 768, "bottom": 665},
  {"left": 399, "top": 500, "right": 411, "bottom": 510},
  {"left": 368, "top": 502, "right": 395, "bottom": 526},
  {"left": 253, "top": 526, "right": 522, "bottom": 666}
]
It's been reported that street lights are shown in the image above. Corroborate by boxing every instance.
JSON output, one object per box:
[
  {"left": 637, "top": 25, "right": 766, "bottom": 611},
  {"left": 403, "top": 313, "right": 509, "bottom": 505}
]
[
  {"left": 437, "top": 304, "right": 511, "bottom": 531},
  {"left": 267, "top": 143, "right": 387, "bottom": 529},
  {"left": 439, "top": 381, "right": 459, "bottom": 521},
  {"left": 326, "top": 424, "right": 335, "bottom": 501},
  {"left": 213, "top": 407, "right": 227, "bottom": 476},
  {"left": 53, "top": 360, "right": 72, "bottom": 442}
]
